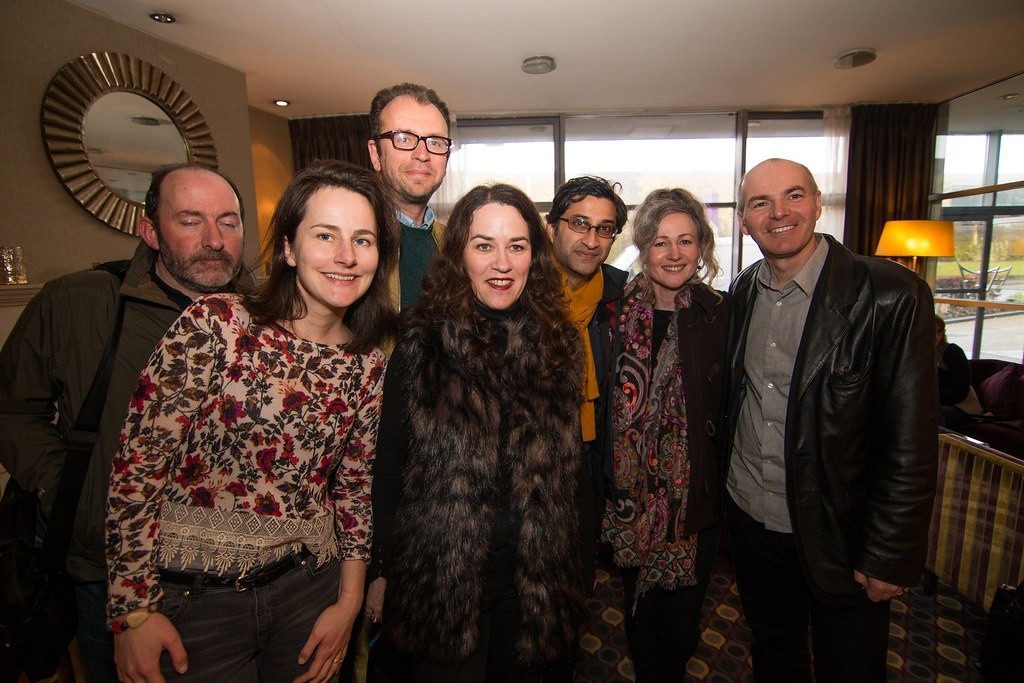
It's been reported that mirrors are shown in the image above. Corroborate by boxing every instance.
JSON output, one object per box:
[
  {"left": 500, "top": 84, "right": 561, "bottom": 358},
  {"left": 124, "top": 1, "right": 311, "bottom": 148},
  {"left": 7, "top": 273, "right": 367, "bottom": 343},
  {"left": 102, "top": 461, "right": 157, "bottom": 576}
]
[{"left": 42, "top": 51, "right": 219, "bottom": 237}]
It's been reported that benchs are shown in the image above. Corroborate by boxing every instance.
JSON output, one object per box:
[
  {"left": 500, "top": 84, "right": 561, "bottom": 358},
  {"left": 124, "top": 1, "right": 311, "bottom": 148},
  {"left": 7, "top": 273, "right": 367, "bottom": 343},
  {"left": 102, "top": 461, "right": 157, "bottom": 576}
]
[{"left": 949, "top": 359, "right": 1024, "bottom": 459}]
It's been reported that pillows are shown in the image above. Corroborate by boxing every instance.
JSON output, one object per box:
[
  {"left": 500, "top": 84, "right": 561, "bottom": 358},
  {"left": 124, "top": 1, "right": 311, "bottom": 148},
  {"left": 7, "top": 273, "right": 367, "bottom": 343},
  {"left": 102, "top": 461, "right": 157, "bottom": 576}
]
[{"left": 980, "top": 363, "right": 1024, "bottom": 421}]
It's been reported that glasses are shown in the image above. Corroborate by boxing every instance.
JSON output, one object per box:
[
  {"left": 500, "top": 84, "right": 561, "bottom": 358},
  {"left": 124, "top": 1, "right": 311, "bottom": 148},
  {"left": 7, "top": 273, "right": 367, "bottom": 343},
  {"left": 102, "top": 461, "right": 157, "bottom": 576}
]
[
  {"left": 555, "top": 217, "right": 619, "bottom": 239},
  {"left": 371, "top": 130, "right": 452, "bottom": 155}
]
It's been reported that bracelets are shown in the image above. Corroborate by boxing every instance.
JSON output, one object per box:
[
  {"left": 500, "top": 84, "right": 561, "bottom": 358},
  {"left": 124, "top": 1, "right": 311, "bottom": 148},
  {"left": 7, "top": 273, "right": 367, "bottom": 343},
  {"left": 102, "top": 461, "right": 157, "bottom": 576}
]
[{"left": 106, "top": 603, "right": 157, "bottom": 633}]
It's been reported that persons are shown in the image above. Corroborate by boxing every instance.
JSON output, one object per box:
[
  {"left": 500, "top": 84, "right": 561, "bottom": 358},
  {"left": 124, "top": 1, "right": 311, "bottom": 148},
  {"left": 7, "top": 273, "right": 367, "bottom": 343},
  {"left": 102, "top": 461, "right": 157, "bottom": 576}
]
[
  {"left": 547, "top": 177, "right": 629, "bottom": 590},
  {"left": 935, "top": 315, "right": 983, "bottom": 415},
  {"left": 102, "top": 160, "right": 396, "bottom": 683},
  {"left": 347, "top": 82, "right": 452, "bottom": 359},
  {"left": 364, "top": 184, "right": 584, "bottom": 683},
  {"left": 0, "top": 167, "right": 255, "bottom": 683},
  {"left": 600, "top": 189, "right": 735, "bottom": 683},
  {"left": 730, "top": 158, "right": 939, "bottom": 683}
]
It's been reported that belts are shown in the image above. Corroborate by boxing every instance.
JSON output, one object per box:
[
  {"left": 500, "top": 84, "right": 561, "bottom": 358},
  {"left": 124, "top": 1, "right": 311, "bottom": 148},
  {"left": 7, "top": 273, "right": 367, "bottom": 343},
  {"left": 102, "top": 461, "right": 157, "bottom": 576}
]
[{"left": 157, "top": 544, "right": 311, "bottom": 593}]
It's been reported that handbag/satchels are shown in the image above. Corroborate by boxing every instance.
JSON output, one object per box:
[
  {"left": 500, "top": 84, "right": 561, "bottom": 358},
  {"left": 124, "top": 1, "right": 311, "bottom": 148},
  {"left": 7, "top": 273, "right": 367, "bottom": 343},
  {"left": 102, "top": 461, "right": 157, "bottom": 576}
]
[
  {"left": 954, "top": 384, "right": 994, "bottom": 416},
  {"left": 0, "top": 474, "right": 78, "bottom": 683}
]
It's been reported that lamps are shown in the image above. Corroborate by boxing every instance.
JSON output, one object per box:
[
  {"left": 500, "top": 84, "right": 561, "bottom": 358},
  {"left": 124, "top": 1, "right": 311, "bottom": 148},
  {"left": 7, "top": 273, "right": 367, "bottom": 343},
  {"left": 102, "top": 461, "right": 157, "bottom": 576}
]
[
  {"left": 522, "top": 56, "right": 557, "bottom": 74},
  {"left": 874, "top": 220, "right": 955, "bottom": 272}
]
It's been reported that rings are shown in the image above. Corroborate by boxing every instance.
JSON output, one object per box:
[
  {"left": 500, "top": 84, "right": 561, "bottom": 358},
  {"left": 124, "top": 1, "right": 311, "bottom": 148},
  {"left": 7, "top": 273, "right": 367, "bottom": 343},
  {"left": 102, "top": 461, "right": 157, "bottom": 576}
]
[{"left": 333, "top": 659, "right": 342, "bottom": 664}]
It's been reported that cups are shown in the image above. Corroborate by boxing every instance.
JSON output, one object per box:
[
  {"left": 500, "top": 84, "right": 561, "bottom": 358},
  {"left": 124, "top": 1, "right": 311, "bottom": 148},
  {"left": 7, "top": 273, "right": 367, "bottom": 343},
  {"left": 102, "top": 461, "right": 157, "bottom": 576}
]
[{"left": 0, "top": 246, "right": 27, "bottom": 285}]
